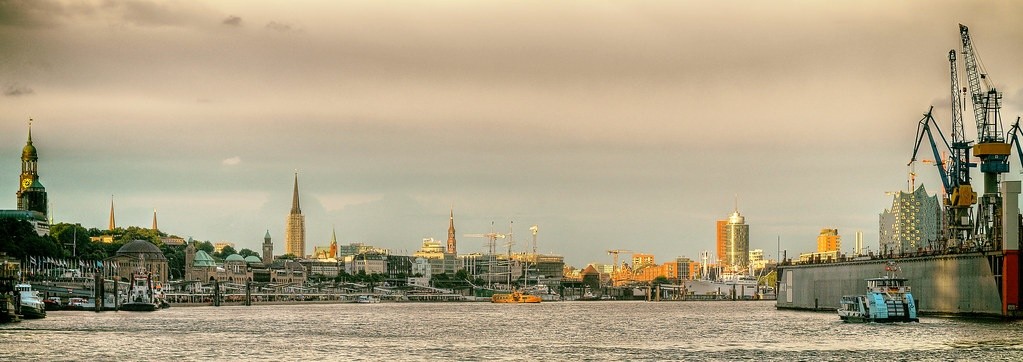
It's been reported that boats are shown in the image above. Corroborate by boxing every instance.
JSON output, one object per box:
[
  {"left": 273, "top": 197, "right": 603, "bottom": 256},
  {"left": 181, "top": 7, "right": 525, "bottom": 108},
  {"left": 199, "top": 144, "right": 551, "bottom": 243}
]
[
  {"left": 120, "top": 270, "right": 157, "bottom": 311},
  {"left": 492, "top": 292, "right": 542, "bottom": 303},
  {"left": 42, "top": 297, "right": 125, "bottom": 311},
  {"left": 14, "top": 284, "right": 46, "bottom": 319},
  {"left": 157, "top": 300, "right": 170, "bottom": 309},
  {"left": 835, "top": 276, "right": 921, "bottom": 324}
]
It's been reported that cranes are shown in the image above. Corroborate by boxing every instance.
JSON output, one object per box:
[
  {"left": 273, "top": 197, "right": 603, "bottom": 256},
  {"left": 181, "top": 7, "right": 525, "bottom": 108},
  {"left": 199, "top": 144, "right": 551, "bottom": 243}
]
[
  {"left": 606, "top": 249, "right": 636, "bottom": 287},
  {"left": 463, "top": 220, "right": 516, "bottom": 253},
  {"left": 957, "top": 22, "right": 1023, "bottom": 175},
  {"left": 529, "top": 224, "right": 538, "bottom": 264},
  {"left": 906, "top": 48, "right": 977, "bottom": 210}
]
